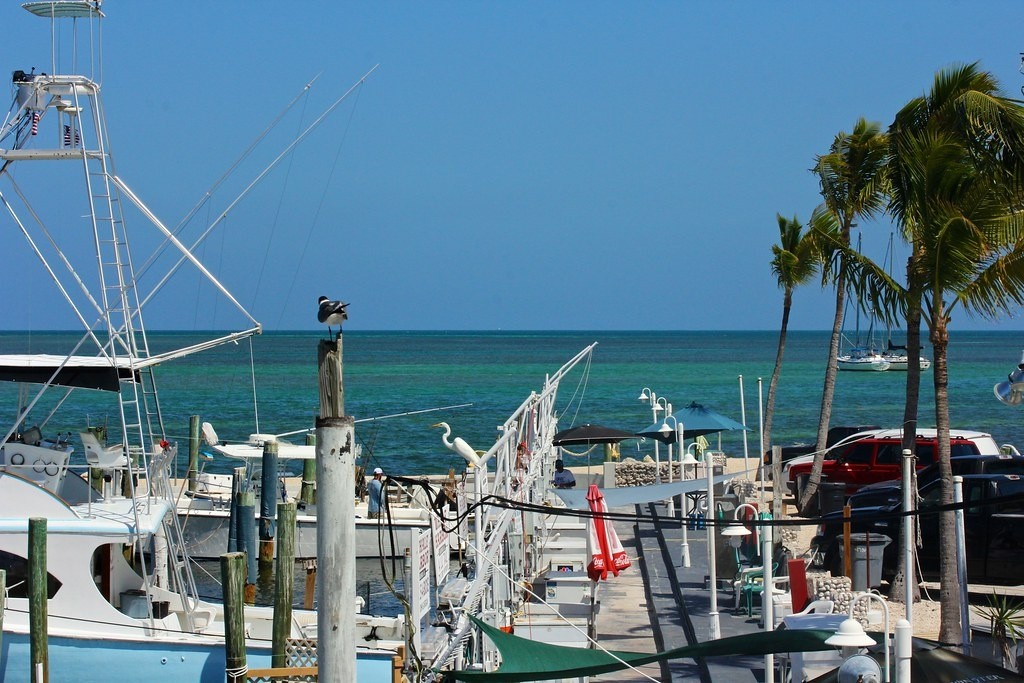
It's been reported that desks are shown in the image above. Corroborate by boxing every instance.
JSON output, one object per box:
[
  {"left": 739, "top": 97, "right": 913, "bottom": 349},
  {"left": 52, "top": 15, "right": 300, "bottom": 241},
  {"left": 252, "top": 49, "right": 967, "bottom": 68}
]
[
  {"left": 215, "top": 444, "right": 317, "bottom": 494},
  {"left": 784, "top": 616, "right": 854, "bottom": 630}
]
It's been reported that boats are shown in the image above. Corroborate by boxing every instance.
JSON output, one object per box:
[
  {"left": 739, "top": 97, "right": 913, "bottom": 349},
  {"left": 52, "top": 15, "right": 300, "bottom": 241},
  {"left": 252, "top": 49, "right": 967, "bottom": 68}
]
[
  {"left": 837, "top": 305, "right": 890, "bottom": 372},
  {"left": 95, "top": 433, "right": 475, "bottom": 561},
  {"left": 994, "top": 350, "right": 1024, "bottom": 407},
  {"left": 876, "top": 331, "right": 931, "bottom": 371},
  {"left": 0, "top": 471, "right": 407, "bottom": 683},
  {"left": 77, "top": 441, "right": 317, "bottom": 507}
]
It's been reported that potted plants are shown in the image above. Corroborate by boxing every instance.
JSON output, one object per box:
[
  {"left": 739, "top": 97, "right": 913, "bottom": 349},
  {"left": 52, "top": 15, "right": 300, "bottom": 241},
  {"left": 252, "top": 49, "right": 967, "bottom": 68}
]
[{"left": 967, "top": 586, "right": 1024, "bottom": 671}]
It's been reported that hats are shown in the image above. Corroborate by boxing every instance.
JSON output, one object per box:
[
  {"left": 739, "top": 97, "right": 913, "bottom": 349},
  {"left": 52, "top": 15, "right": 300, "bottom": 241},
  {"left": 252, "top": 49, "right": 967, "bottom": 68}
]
[
  {"left": 373, "top": 468, "right": 383, "bottom": 474},
  {"left": 555, "top": 460, "right": 563, "bottom": 467}
]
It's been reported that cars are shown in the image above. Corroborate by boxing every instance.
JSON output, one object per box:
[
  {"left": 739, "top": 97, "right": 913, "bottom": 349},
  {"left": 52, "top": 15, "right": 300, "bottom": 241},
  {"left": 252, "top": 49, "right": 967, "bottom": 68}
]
[
  {"left": 760, "top": 426, "right": 882, "bottom": 466},
  {"left": 808, "top": 473, "right": 1024, "bottom": 585},
  {"left": 847, "top": 454, "right": 1024, "bottom": 508}
]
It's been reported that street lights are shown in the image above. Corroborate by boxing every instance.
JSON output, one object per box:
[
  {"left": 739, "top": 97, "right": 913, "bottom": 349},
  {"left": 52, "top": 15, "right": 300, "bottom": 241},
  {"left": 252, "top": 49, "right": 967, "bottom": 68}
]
[
  {"left": 721, "top": 503, "right": 774, "bottom": 683},
  {"left": 680, "top": 443, "right": 721, "bottom": 640},
  {"left": 651, "top": 397, "right": 675, "bottom": 518},
  {"left": 657, "top": 415, "right": 691, "bottom": 567},
  {"left": 823, "top": 593, "right": 913, "bottom": 683},
  {"left": 638, "top": 387, "right": 660, "bottom": 486}
]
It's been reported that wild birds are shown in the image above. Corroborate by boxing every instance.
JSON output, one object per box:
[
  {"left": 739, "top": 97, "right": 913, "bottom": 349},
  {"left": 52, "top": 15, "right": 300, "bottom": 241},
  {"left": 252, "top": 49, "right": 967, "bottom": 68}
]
[
  {"left": 427, "top": 422, "right": 483, "bottom": 469},
  {"left": 317, "top": 296, "right": 351, "bottom": 341}
]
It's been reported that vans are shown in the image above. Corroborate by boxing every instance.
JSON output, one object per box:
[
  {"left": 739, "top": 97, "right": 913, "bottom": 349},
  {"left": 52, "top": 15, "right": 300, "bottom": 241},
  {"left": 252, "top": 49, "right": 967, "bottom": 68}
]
[{"left": 784, "top": 427, "right": 1000, "bottom": 514}]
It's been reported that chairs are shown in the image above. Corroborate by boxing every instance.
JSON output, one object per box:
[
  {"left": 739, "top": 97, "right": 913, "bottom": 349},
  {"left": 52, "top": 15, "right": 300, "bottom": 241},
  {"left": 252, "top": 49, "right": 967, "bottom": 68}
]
[
  {"left": 727, "top": 554, "right": 787, "bottom": 627},
  {"left": 787, "top": 599, "right": 835, "bottom": 616},
  {"left": 80, "top": 431, "right": 132, "bottom": 503},
  {"left": 105, "top": 444, "right": 134, "bottom": 500}
]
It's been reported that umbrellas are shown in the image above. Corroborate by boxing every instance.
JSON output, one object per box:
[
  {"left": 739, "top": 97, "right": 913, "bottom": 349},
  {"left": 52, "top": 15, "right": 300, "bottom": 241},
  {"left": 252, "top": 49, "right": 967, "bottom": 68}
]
[
  {"left": 637, "top": 402, "right": 752, "bottom": 479},
  {"left": 586, "top": 485, "right": 631, "bottom": 649},
  {"left": 552, "top": 424, "right": 639, "bottom": 485}
]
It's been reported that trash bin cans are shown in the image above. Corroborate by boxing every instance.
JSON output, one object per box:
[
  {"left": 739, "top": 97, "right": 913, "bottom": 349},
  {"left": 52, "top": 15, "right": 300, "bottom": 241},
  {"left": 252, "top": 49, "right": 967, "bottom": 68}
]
[{"left": 836, "top": 531, "right": 893, "bottom": 596}]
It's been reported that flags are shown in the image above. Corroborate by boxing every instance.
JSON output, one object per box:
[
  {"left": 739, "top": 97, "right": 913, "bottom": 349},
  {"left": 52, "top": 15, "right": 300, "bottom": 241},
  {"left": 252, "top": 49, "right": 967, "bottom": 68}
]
[{"left": 64, "top": 125, "right": 80, "bottom": 146}]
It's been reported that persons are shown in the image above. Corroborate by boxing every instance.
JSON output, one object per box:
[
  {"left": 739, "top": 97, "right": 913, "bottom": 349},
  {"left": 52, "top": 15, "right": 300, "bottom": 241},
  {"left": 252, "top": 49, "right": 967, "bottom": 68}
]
[
  {"left": 367, "top": 467, "right": 384, "bottom": 519},
  {"left": 555, "top": 460, "right": 576, "bottom": 489},
  {"left": 410, "top": 475, "right": 429, "bottom": 507}
]
